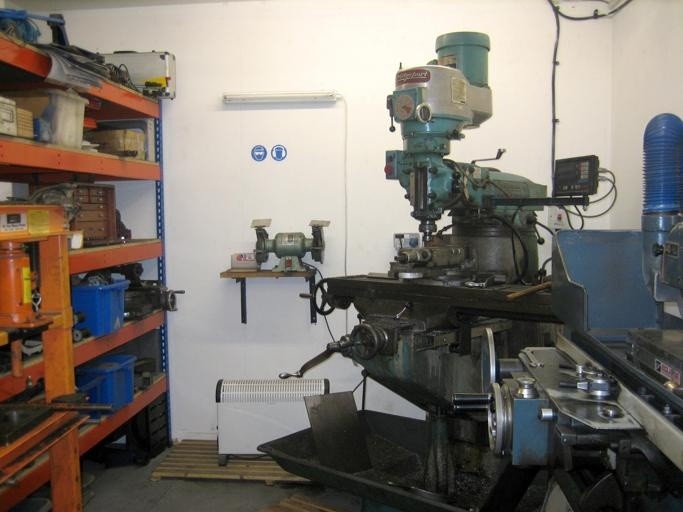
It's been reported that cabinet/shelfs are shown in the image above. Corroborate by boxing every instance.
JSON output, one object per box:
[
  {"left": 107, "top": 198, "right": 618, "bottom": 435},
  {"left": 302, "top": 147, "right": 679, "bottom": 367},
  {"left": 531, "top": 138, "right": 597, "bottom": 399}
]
[{"left": 0, "top": 29, "right": 177, "bottom": 511}]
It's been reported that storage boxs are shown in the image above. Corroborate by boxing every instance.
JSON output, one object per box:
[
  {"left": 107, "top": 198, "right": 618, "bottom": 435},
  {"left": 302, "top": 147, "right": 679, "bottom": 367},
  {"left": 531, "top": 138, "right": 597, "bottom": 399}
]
[
  {"left": 74, "top": 353, "right": 137, "bottom": 418},
  {"left": 68, "top": 277, "right": 129, "bottom": 336}
]
